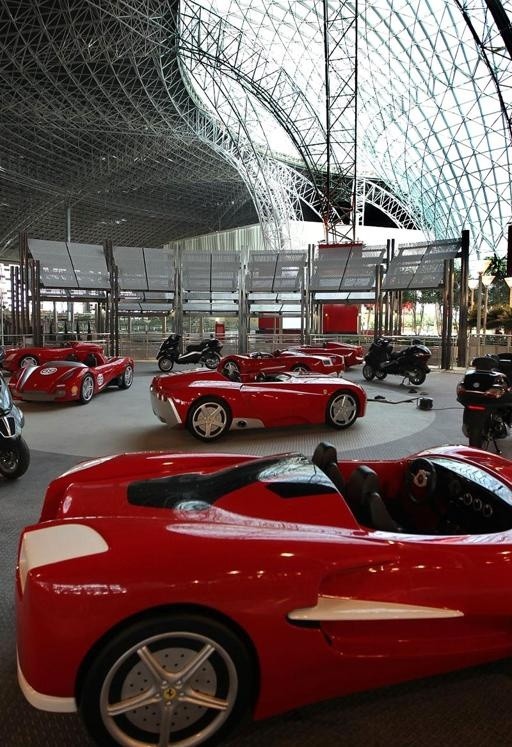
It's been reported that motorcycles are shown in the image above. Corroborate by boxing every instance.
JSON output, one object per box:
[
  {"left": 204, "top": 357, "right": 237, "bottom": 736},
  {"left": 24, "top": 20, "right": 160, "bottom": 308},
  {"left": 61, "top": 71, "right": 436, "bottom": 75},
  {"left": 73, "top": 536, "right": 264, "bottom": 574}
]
[
  {"left": 156, "top": 333, "right": 223, "bottom": 372},
  {"left": 0, "top": 370, "right": 31, "bottom": 479},
  {"left": 362, "top": 336, "right": 432, "bottom": 385},
  {"left": 457, "top": 352, "right": 512, "bottom": 454}
]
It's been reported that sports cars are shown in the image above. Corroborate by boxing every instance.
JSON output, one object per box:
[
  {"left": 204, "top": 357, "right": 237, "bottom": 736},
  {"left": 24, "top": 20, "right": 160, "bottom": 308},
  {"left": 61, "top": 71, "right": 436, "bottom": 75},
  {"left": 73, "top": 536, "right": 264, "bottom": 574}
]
[
  {"left": 14, "top": 442, "right": 512, "bottom": 747},
  {"left": 150, "top": 342, "right": 367, "bottom": 443},
  {"left": 0, "top": 341, "right": 136, "bottom": 404}
]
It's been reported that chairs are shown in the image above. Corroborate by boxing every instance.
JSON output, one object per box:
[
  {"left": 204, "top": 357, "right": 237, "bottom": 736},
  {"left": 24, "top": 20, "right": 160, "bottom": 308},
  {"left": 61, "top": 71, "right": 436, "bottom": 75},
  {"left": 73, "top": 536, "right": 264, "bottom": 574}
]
[
  {"left": 65, "top": 351, "right": 98, "bottom": 368},
  {"left": 311, "top": 440, "right": 399, "bottom": 533},
  {"left": 221, "top": 367, "right": 242, "bottom": 381},
  {"left": 56, "top": 342, "right": 71, "bottom": 348}
]
